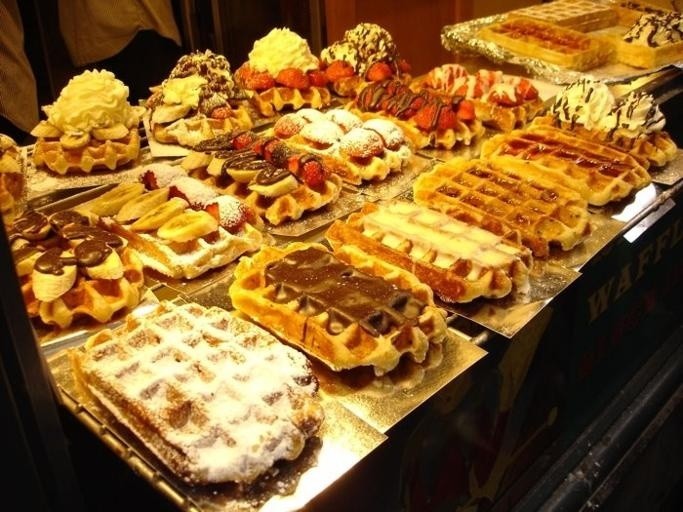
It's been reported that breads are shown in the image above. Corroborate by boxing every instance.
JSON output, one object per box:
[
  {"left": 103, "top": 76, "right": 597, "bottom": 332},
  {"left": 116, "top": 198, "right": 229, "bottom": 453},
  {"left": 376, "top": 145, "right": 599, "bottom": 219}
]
[{"left": 0, "top": 1, "right": 683, "bottom": 497}]
